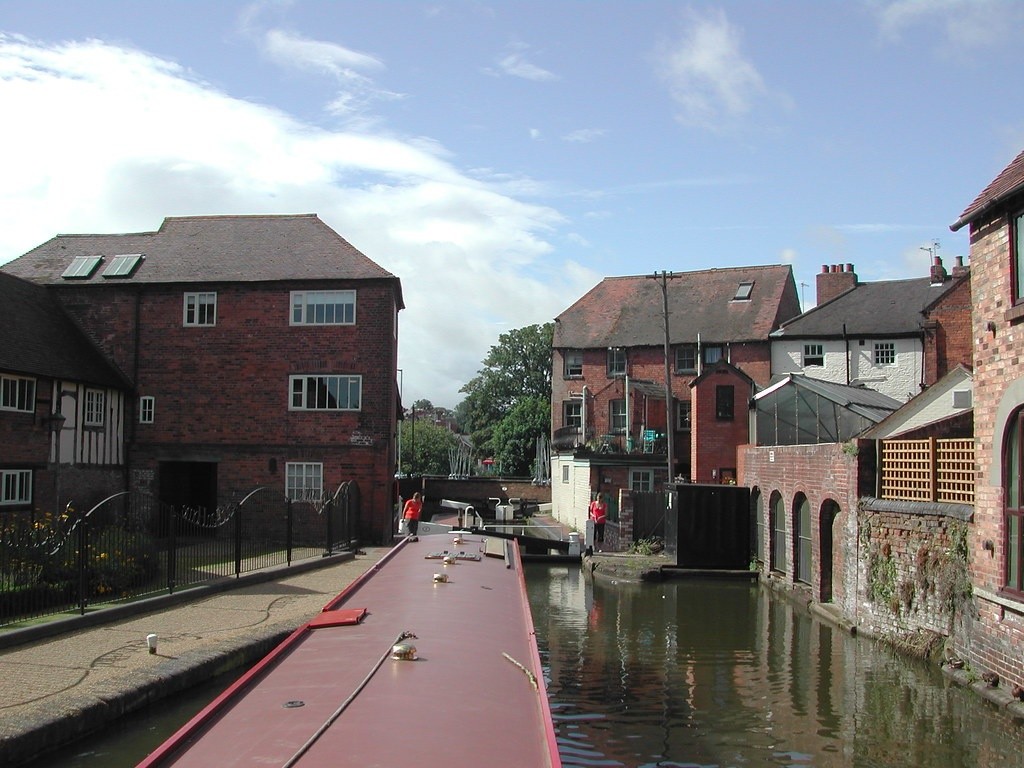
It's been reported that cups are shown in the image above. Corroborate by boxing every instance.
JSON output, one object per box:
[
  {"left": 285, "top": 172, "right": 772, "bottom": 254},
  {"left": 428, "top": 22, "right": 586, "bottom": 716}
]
[{"left": 146, "top": 634, "right": 158, "bottom": 654}]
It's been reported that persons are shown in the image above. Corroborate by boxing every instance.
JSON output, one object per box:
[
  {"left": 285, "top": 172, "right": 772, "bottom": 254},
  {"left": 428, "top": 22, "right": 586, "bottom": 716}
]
[
  {"left": 590, "top": 492, "right": 607, "bottom": 553},
  {"left": 401, "top": 492, "right": 422, "bottom": 535}
]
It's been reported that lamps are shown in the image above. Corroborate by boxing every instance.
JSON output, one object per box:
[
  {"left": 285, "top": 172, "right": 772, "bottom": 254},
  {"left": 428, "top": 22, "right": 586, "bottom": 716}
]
[
  {"left": 982, "top": 321, "right": 995, "bottom": 331},
  {"left": 747, "top": 398, "right": 756, "bottom": 410}
]
